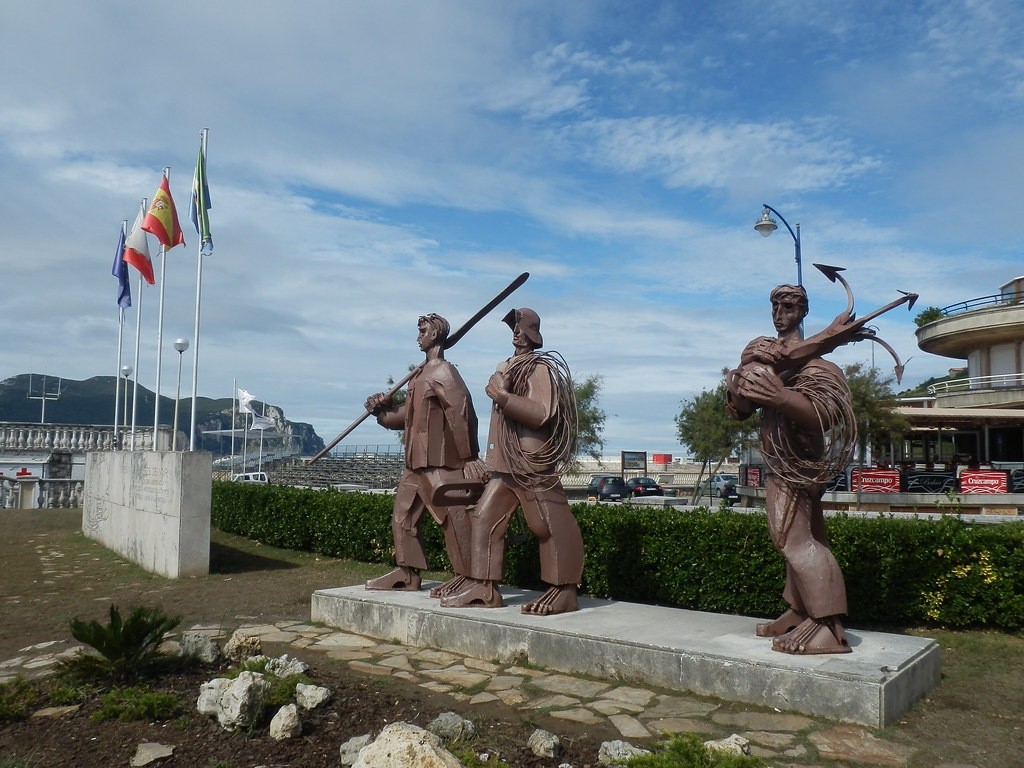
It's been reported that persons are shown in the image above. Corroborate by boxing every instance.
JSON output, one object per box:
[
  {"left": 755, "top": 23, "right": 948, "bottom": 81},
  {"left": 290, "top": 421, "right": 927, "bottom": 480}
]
[
  {"left": 441, "top": 307, "right": 586, "bottom": 615},
  {"left": 364, "top": 314, "right": 488, "bottom": 601},
  {"left": 727, "top": 284, "right": 852, "bottom": 655}
]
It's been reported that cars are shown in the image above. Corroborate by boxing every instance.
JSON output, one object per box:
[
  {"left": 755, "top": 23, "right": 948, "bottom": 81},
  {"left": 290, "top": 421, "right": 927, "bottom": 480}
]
[
  {"left": 720, "top": 477, "right": 744, "bottom": 507},
  {"left": 699, "top": 474, "right": 740, "bottom": 497},
  {"left": 587, "top": 476, "right": 627, "bottom": 502},
  {"left": 624, "top": 477, "right": 664, "bottom": 496}
]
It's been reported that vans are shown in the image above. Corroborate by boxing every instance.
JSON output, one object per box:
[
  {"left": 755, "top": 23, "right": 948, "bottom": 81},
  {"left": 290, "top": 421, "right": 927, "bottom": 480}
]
[{"left": 230, "top": 472, "right": 268, "bottom": 483}]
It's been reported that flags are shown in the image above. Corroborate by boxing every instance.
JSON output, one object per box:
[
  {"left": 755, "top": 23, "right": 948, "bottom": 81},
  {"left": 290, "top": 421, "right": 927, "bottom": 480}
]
[
  {"left": 237, "top": 388, "right": 256, "bottom": 414},
  {"left": 112, "top": 227, "right": 131, "bottom": 309},
  {"left": 141, "top": 175, "right": 186, "bottom": 258},
  {"left": 251, "top": 409, "right": 276, "bottom": 429},
  {"left": 123, "top": 206, "right": 155, "bottom": 285},
  {"left": 189, "top": 139, "right": 214, "bottom": 256}
]
